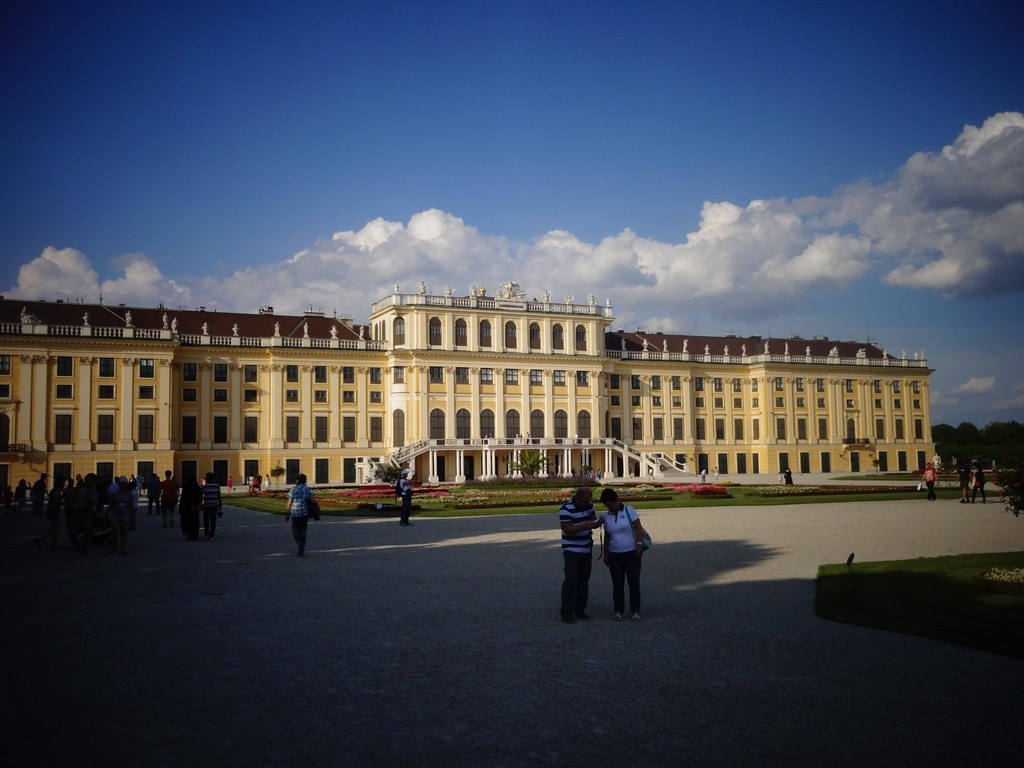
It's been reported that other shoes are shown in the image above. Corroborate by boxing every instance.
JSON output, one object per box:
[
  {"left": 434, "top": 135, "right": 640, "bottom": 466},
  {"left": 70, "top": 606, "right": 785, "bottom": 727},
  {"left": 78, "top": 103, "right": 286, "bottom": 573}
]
[
  {"left": 161, "top": 524, "right": 167, "bottom": 529},
  {"left": 562, "top": 614, "right": 574, "bottom": 623},
  {"left": 48, "top": 546, "right": 58, "bottom": 551},
  {"left": 110, "top": 545, "right": 119, "bottom": 554},
  {"left": 128, "top": 527, "right": 136, "bottom": 531},
  {"left": 35, "top": 539, "right": 41, "bottom": 549},
  {"left": 574, "top": 611, "right": 589, "bottom": 621},
  {"left": 401, "top": 521, "right": 414, "bottom": 525},
  {"left": 80, "top": 553, "right": 88, "bottom": 557},
  {"left": 206, "top": 535, "right": 216, "bottom": 540}
]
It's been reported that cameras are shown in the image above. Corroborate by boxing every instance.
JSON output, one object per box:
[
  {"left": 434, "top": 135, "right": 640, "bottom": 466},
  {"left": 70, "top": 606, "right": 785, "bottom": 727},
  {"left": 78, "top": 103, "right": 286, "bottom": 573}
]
[
  {"left": 593, "top": 517, "right": 601, "bottom": 525},
  {"left": 217, "top": 511, "right": 223, "bottom": 518}
]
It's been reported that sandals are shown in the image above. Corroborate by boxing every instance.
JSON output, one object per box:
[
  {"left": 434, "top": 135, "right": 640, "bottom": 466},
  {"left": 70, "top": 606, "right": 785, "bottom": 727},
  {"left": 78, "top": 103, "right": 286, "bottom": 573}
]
[
  {"left": 632, "top": 613, "right": 641, "bottom": 622},
  {"left": 615, "top": 611, "right": 622, "bottom": 622}
]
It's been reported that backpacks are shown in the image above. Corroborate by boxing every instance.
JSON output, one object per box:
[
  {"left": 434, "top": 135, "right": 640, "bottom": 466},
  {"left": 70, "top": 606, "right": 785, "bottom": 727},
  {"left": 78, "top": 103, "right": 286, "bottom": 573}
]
[{"left": 395, "top": 478, "right": 407, "bottom": 497}]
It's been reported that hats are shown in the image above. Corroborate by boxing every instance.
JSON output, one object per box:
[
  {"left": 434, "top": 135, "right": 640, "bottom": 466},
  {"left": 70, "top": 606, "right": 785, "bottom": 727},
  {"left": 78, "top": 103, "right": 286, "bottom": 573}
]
[
  {"left": 118, "top": 476, "right": 127, "bottom": 483},
  {"left": 401, "top": 471, "right": 410, "bottom": 475}
]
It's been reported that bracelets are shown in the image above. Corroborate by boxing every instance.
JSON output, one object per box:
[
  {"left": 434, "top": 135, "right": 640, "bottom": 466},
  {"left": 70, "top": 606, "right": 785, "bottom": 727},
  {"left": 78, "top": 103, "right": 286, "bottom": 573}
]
[{"left": 637, "top": 542, "right": 642, "bottom": 545}]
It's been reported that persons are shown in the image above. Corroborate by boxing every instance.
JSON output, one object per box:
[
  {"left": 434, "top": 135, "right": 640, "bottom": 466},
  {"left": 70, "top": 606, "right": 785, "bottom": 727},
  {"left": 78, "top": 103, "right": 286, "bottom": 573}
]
[
  {"left": 35, "top": 473, "right": 139, "bottom": 557},
  {"left": 600, "top": 488, "right": 643, "bottom": 622},
  {"left": 921, "top": 462, "right": 938, "bottom": 501},
  {"left": 480, "top": 284, "right": 486, "bottom": 297},
  {"left": 228, "top": 476, "right": 232, "bottom": 488},
  {"left": 597, "top": 468, "right": 602, "bottom": 480},
  {"left": 543, "top": 289, "right": 610, "bottom": 307},
  {"left": 83, "top": 313, "right": 91, "bottom": 326},
  {"left": 246, "top": 473, "right": 271, "bottom": 492},
  {"left": 778, "top": 468, "right": 793, "bottom": 484},
  {"left": 506, "top": 281, "right": 514, "bottom": 298},
  {"left": 420, "top": 281, "right": 425, "bottom": 294},
  {"left": 359, "top": 324, "right": 364, "bottom": 338},
  {"left": 701, "top": 467, "right": 706, "bottom": 483},
  {"left": 572, "top": 468, "right": 575, "bottom": 476},
  {"left": 285, "top": 474, "right": 315, "bottom": 555},
  {"left": 275, "top": 322, "right": 280, "bottom": 337},
  {"left": 648, "top": 467, "right": 656, "bottom": 481},
  {"left": 621, "top": 337, "right": 928, "bottom": 361},
  {"left": 399, "top": 470, "right": 412, "bottom": 526},
  {"left": 304, "top": 323, "right": 309, "bottom": 335},
  {"left": 714, "top": 466, "right": 719, "bottom": 481},
  {"left": 559, "top": 486, "right": 606, "bottom": 623},
  {"left": 525, "top": 432, "right": 531, "bottom": 444},
  {"left": 125, "top": 311, "right": 133, "bottom": 324},
  {"left": 135, "top": 469, "right": 223, "bottom": 541},
  {"left": 628, "top": 466, "right": 632, "bottom": 478},
  {"left": 3, "top": 473, "right": 48, "bottom": 517},
  {"left": 163, "top": 313, "right": 178, "bottom": 330},
  {"left": 955, "top": 460, "right": 986, "bottom": 504}
]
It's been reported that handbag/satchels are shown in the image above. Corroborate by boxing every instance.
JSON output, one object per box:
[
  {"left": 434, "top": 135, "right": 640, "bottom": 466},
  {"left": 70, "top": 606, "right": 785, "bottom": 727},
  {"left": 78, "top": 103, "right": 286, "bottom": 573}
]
[
  {"left": 625, "top": 505, "right": 651, "bottom": 552},
  {"left": 191, "top": 497, "right": 204, "bottom": 512},
  {"left": 306, "top": 487, "right": 315, "bottom": 518}
]
